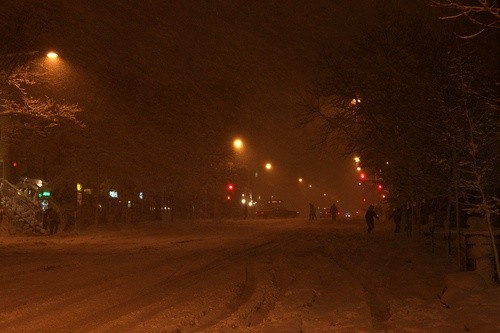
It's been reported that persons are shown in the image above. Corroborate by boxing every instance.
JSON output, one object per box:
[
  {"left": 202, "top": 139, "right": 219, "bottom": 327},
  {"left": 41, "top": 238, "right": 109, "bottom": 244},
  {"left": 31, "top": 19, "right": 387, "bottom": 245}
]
[
  {"left": 389, "top": 205, "right": 403, "bottom": 233},
  {"left": 309, "top": 202, "right": 339, "bottom": 221},
  {"left": 365, "top": 205, "right": 380, "bottom": 234},
  {"left": 42, "top": 205, "right": 60, "bottom": 234}
]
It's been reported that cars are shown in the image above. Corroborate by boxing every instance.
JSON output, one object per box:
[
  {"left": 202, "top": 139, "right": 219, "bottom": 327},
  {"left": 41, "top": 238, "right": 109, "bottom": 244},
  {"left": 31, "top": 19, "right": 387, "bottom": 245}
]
[
  {"left": 319, "top": 207, "right": 340, "bottom": 218},
  {"left": 344, "top": 211, "right": 351, "bottom": 218},
  {"left": 255, "top": 200, "right": 299, "bottom": 219}
]
